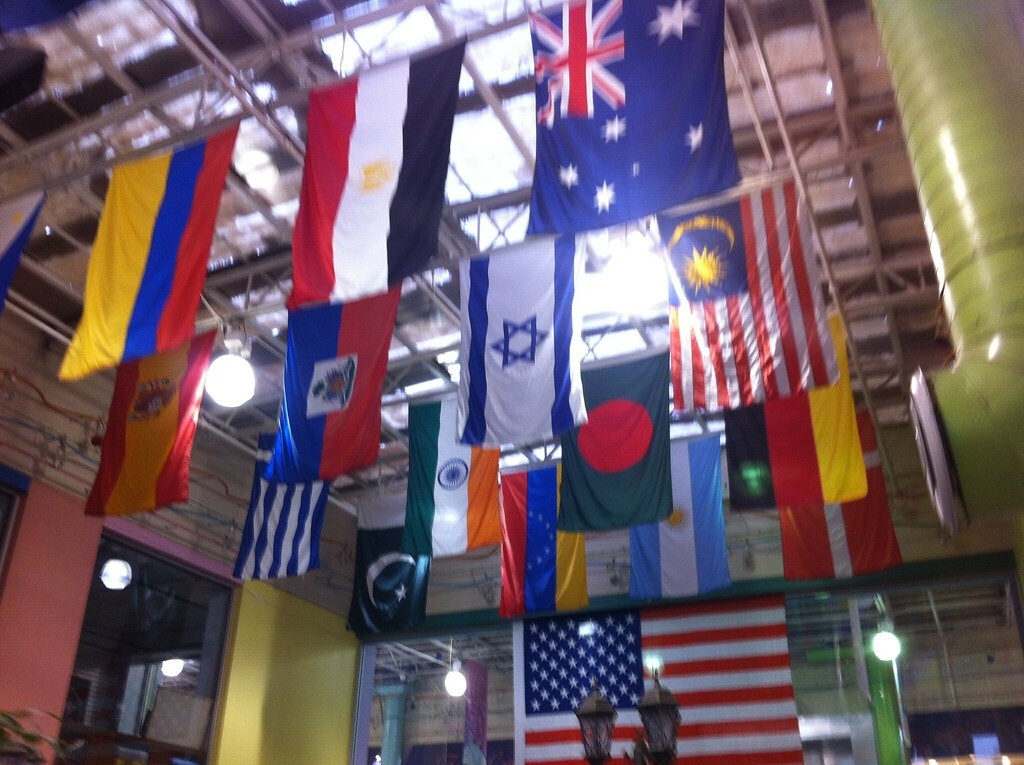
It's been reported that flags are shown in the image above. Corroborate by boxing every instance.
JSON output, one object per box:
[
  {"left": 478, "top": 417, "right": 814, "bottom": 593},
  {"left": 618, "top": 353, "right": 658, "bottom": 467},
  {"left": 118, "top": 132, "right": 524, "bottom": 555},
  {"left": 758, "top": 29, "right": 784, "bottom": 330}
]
[
  {"left": 766, "top": 314, "right": 868, "bottom": 504},
  {"left": 58, "top": 120, "right": 242, "bottom": 381},
  {"left": 512, "top": 596, "right": 805, "bottom": 765},
  {"left": 628, "top": 432, "right": 733, "bottom": 599},
  {"left": 85, "top": 328, "right": 217, "bottom": 518},
  {"left": 267, "top": 291, "right": 399, "bottom": 481},
  {"left": 0, "top": 192, "right": 48, "bottom": 308},
  {"left": 774, "top": 406, "right": 902, "bottom": 579},
  {"left": 234, "top": 433, "right": 329, "bottom": 580},
  {"left": 285, "top": 38, "right": 468, "bottom": 307},
  {"left": 658, "top": 173, "right": 840, "bottom": 425},
  {"left": 454, "top": 232, "right": 589, "bottom": 445},
  {"left": 526, "top": 0, "right": 739, "bottom": 237},
  {"left": 500, "top": 459, "right": 588, "bottom": 616},
  {"left": 399, "top": 396, "right": 504, "bottom": 555},
  {"left": 347, "top": 486, "right": 429, "bottom": 633},
  {"left": 557, "top": 348, "right": 673, "bottom": 532}
]
